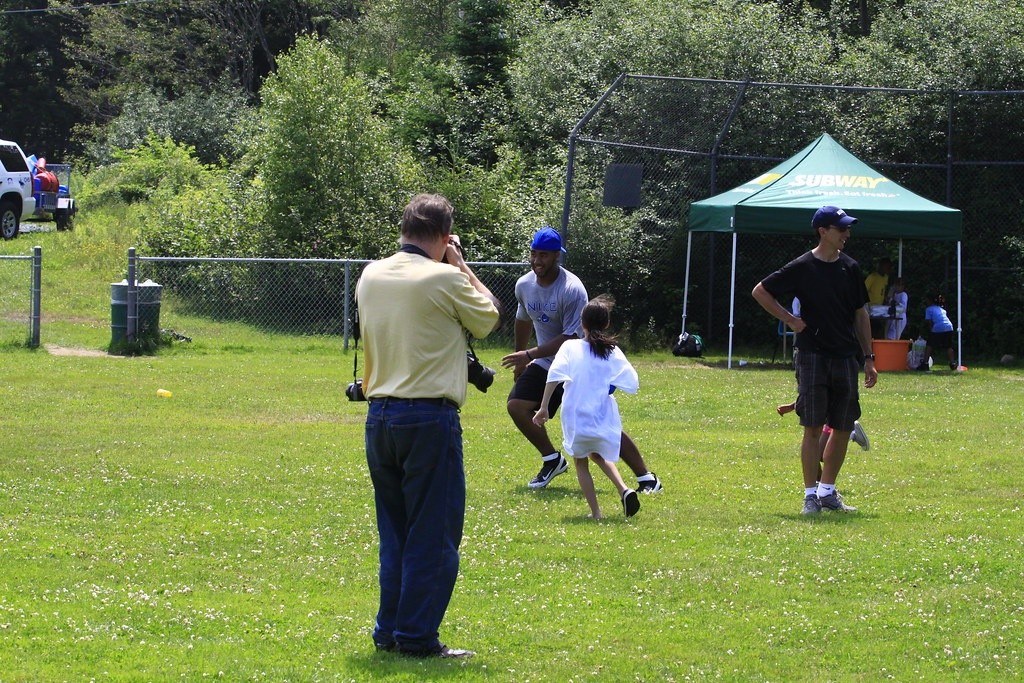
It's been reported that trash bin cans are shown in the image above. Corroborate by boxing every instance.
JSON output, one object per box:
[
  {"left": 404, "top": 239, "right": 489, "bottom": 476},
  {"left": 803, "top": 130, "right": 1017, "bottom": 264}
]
[{"left": 111, "top": 283, "right": 163, "bottom": 338}]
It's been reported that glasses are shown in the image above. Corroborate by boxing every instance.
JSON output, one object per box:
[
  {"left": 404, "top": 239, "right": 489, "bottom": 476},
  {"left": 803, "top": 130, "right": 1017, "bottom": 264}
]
[{"left": 824, "top": 225, "right": 852, "bottom": 232}]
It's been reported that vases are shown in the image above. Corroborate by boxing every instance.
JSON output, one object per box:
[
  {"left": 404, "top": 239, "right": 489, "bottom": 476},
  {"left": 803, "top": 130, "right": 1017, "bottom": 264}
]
[{"left": 872, "top": 339, "right": 913, "bottom": 371}]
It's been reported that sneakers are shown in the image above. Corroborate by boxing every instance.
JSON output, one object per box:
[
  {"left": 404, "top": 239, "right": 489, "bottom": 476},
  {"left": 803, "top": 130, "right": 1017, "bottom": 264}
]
[
  {"left": 635, "top": 472, "right": 663, "bottom": 493},
  {"left": 528, "top": 451, "right": 567, "bottom": 488},
  {"left": 852, "top": 420, "right": 868, "bottom": 450},
  {"left": 820, "top": 490, "right": 854, "bottom": 510},
  {"left": 803, "top": 494, "right": 821, "bottom": 512}
]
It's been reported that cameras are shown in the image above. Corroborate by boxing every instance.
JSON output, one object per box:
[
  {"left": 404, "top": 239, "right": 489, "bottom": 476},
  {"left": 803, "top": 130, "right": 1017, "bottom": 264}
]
[
  {"left": 465, "top": 352, "right": 496, "bottom": 393},
  {"left": 346, "top": 379, "right": 367, "bottom": 401}
]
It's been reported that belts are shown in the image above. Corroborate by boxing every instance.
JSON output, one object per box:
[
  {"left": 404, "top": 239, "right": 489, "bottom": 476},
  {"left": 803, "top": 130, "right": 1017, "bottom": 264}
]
[{"left": 371, "top": 396, "right": 459, "bottom": 409}]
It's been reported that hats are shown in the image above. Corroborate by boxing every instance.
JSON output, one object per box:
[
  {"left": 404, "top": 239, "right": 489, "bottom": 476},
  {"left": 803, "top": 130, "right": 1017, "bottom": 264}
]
[
  {"left": 812, "top": 205, "right": 859, "bottom": 226},
  {"left": 532, "top": 227, "right": 566, "bottom": 253}
]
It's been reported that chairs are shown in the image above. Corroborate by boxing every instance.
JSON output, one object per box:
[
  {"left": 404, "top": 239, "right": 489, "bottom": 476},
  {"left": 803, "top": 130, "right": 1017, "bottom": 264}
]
[{"left": 771, "top": 308, "right": 797, "bottom": 364}]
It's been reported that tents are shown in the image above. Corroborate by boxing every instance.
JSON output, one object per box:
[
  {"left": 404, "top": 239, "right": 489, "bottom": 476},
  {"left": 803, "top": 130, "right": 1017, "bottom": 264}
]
[{"left": 682, "top": 133, "right": 963, "bottom": 371}]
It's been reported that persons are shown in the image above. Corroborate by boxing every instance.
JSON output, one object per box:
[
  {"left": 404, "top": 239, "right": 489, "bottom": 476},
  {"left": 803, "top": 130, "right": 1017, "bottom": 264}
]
[
  {"left": 916, "top": 293, "right": 958, "bottom": 371},
  {"left": 532, "top": 293, "right": 640, "bottom": 519},
  {"left": 777, "top": 400, "right": 869, "bottom": 487},
  {"left": 500, "top": 227, "right": 664, "bottom": 495},
  {"left": 354, "top": 193, "right": 504, "bottom": 659},
  {"left": 752, "top": 206, "right": 878, "bottom": 513},
  {"left": 883, "top": 278, "right": 908, "bottom": 340},
  {"left": 864, "top": 256, "right": 892, "bottom": 339}
]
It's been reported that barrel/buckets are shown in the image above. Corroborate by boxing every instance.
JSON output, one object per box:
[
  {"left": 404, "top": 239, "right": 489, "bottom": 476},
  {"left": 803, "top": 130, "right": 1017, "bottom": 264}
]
[{"left": 872, "top": 339, "right": 911, "bottom": 372}]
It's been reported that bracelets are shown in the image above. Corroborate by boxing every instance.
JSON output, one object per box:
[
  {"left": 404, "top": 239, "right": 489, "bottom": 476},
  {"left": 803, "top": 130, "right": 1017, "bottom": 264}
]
[{"left": 526, "top": 351, "right": 533, "bottom": 360}]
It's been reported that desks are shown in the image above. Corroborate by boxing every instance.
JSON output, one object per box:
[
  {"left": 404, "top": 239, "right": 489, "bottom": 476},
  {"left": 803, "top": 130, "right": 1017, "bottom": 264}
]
[{"left": 869, "top": 317, "right": 904, "bottom": 341}]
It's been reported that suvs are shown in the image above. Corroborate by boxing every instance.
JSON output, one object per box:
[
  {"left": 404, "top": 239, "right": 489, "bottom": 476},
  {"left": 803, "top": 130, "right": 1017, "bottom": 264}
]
[{"left": 0, "top": 139, "right": 36, "bottom": 241}]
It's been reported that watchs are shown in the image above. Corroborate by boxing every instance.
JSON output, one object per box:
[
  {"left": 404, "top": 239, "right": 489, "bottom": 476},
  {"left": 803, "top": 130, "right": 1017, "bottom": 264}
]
[{"left": 864, "top": 353, "right": 875, "bottom": 361}]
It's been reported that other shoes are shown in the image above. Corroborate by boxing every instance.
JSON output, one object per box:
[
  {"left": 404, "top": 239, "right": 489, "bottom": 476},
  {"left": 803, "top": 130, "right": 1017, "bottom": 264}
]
[
  {"left": 950, "top": 361, "right": 958, "bottom": 369},
  {"left": 621, "top": 490, "right": 641, "bottom": 518},
  {"left": 916, "top": 363, "right": 929, "bottom": 371},
  {"left": 397, "top": 643, "right": 475, "bottom": 659},
  {"left": 374, "top": 639, "right": 397, "bottom": 653}
]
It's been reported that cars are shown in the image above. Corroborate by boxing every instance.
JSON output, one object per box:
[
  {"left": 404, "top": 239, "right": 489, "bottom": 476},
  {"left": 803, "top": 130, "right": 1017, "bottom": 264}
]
[{"left": 26, "top": 154, "right": 75, "bottom": 231}]
